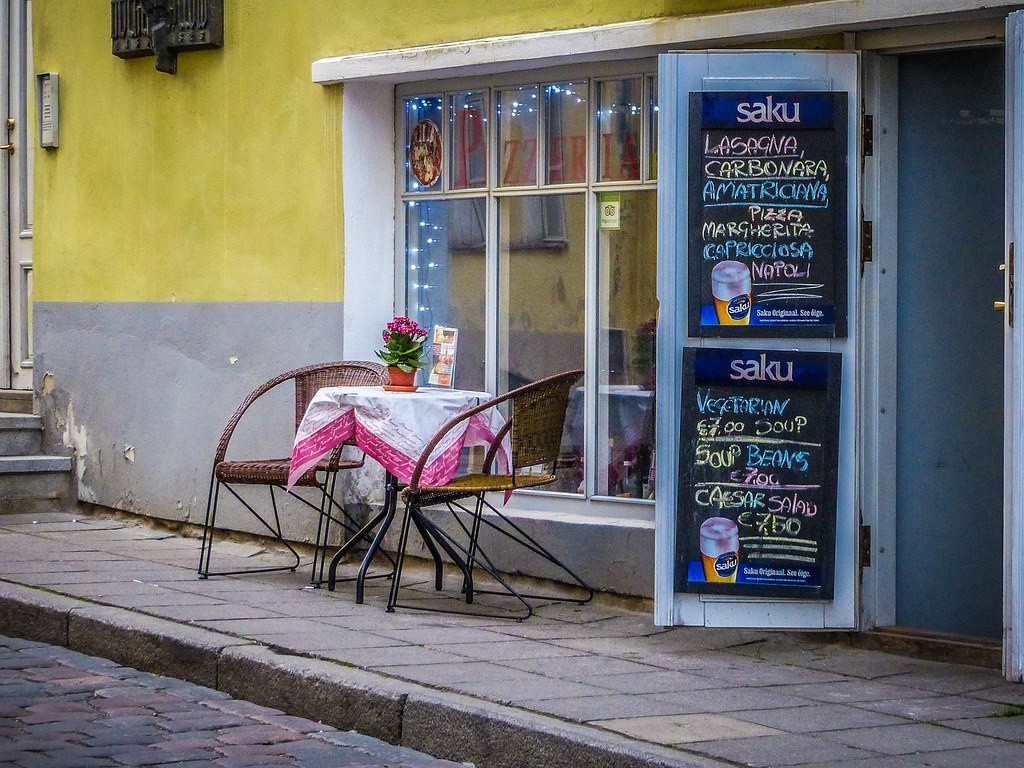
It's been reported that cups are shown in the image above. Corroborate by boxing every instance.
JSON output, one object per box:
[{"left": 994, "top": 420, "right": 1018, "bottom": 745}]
[
  {"left": 711, "top": 260, "right": 752, "bottom": 325},
  {"left": 700, "top": 516, "right": 739, "bottom": 583}
]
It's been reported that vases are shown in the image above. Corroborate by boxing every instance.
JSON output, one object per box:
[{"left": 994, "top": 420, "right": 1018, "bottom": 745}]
[
  {"left": 640, "top": 372, "right": 655, "bottom": 390},
  {"left": 387, "top": 365, "right": 417, "bottom": 386}
]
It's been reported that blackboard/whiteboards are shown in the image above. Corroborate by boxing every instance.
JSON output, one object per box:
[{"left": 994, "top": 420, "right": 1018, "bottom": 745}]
[
  {"left": 672, "top": 347, "right": 843, "bottom": 600},
  {"left": 688, "top": 91, "right": 848, "bottom": 339}
]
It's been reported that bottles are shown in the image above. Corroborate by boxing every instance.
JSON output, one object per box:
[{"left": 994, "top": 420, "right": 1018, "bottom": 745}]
[{"left": 616, "top": 461, "right": 642, "bottom": 499}]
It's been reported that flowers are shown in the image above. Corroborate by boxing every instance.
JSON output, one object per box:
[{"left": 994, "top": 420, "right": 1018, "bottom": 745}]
[
  {"left": 374, "top": 317, "right": 429, "bottom": 374},
  {"left": 630, "top": 319, "right": 658, "bottom": 372}
]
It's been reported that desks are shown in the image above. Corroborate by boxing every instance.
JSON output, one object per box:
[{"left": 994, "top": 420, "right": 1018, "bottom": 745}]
[
  {"left": 567, "top": 386, "right": 652, "bottom": 498},
  {"left": 286, "top": 386, "right": 514, "bottom": 604}
]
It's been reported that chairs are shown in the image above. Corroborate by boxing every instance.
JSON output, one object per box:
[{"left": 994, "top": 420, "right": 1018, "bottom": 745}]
[
  {"left": 384, "top": 369, "right": 593, "bottom": 623},
  {"left": 198, "top": 360, "right": 396, "bottom": 589}
]
